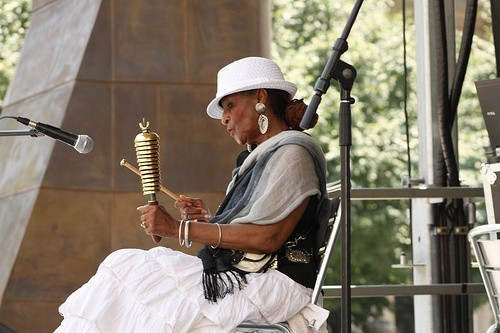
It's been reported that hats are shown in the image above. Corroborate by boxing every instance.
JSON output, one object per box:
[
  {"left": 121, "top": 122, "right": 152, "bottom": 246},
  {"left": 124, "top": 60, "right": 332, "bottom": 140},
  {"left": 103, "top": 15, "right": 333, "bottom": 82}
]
[{"left": 206, "top": 57, "right": 297, "bottom": 119}]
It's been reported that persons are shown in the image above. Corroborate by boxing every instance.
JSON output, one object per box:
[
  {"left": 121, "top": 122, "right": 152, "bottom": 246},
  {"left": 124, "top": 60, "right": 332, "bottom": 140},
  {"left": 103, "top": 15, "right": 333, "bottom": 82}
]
[{"left": 51, "top": 56, "right": 327, "bottom": 333}]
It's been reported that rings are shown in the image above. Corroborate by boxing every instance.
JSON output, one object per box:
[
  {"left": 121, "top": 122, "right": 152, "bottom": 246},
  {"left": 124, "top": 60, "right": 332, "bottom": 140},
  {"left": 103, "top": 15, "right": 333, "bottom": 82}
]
[{"left": 140, "top": 221, "right": 147, "bottom": 229}]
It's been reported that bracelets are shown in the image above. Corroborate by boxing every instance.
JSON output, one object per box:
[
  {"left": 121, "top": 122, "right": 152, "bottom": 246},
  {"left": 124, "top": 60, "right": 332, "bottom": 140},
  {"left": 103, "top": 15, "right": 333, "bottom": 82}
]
[
  {"left": 178, "top": 219, "right": 193, "bottom": 248},
  {"left": 210, "top": 222, "right": 222, "bottom": 249}
]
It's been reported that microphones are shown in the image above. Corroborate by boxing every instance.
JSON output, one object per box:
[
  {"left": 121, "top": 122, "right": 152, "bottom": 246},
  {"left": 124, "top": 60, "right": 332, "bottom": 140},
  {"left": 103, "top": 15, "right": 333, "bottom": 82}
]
[{"left": 17, "top": 116, "right": 94, "bottom": 153}]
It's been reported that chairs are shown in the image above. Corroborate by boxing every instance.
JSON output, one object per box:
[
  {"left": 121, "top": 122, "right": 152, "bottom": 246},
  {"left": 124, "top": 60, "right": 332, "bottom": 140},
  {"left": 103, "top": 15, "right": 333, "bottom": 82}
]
[
  {"left": 238, "top": 180, "right": 341, "bottom": 333},
  {"left": 467, "top": 224, "right": 500, "bottom": 333}
]
